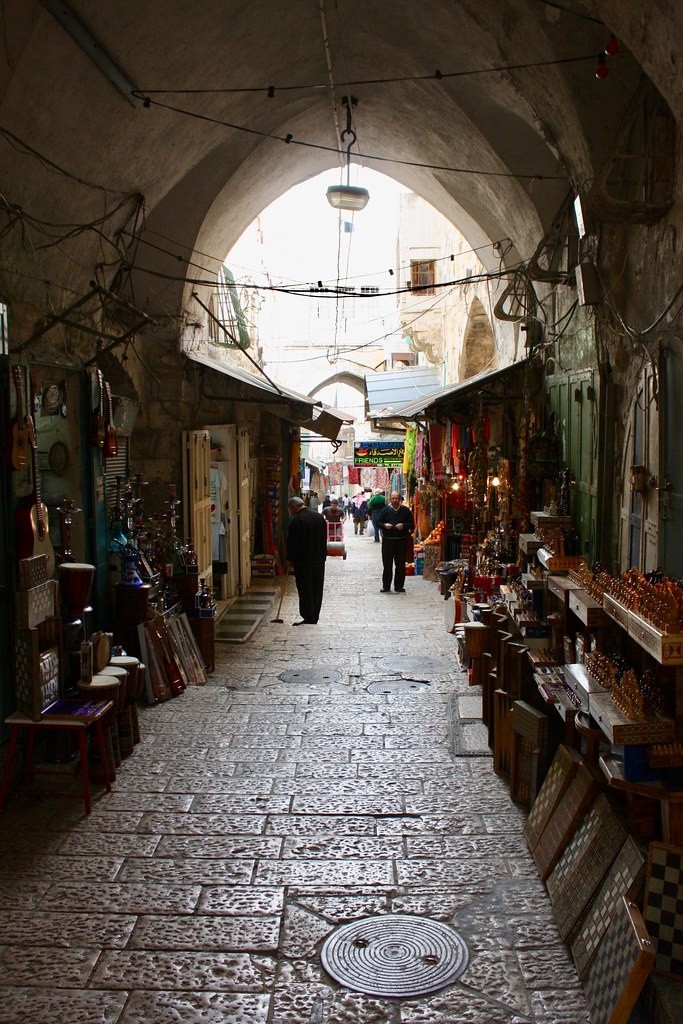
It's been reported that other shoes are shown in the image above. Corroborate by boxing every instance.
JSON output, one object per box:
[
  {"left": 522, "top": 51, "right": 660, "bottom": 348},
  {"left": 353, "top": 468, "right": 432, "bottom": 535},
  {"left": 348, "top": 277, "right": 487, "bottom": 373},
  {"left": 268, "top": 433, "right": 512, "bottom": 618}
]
[
  {"left": 360, "top": 532, "right": 363, "bottom": 535},
  {"left": 394, "top": 588, "right": 405, "bottom": 592},
  {"left": 355, "top": 530, "right": 357, "bottom": 534},
  {"left": 381, "top": 589, "right": 390, "bottom": 592}
]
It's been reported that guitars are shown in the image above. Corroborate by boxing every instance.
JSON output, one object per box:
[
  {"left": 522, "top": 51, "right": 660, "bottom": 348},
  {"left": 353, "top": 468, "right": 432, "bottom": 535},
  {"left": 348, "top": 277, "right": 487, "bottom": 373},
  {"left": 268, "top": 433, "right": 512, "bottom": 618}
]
[
  {"left": 3, "top": 362, "right": 31, "bottom": 472},
  {"left": 92, "top": 369, "right": 119, "bottom": 458},
  {"left": 15, "top": 415, "right": 55, "bottom": 580}
]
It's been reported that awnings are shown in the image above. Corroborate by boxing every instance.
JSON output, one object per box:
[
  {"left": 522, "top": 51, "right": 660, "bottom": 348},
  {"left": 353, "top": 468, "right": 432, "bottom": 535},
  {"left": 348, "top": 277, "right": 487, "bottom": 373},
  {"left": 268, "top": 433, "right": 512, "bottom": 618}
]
[
  {"left": 188, "top": 350, "right": 356, "bottom": 474},
  {"left": 363, "top": 354, "right": 533, "bottom": 422}
]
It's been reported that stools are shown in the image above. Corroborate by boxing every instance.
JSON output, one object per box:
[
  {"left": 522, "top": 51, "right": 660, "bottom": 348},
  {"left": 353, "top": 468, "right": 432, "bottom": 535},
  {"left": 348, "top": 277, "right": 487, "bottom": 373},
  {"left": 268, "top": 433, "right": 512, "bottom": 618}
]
[{"left": 0, "top": 701, "right": 111, "bottom": 814}]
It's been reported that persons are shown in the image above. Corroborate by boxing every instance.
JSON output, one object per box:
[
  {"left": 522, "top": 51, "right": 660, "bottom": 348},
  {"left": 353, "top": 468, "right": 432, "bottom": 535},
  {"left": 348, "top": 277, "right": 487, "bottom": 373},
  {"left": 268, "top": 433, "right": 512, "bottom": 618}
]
[
  {"left": 286, "top": 497, "right": 328, "bottom": 625},
  {"left": 306, "top": 485, "right": 405, "bottom": 542},
  {"left": 375, "top": 491, "right": 414, "bottom": 593}
]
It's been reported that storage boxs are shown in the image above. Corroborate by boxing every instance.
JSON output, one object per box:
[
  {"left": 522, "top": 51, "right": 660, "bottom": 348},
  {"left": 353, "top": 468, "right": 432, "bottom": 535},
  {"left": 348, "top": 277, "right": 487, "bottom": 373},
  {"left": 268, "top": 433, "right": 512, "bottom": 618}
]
[{"left": 464, "top": 505, "right": 683, "bottom": 1024}]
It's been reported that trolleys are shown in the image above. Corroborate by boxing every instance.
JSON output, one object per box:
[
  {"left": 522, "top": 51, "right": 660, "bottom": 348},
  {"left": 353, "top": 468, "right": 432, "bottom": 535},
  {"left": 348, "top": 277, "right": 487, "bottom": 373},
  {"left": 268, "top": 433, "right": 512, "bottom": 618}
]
[{"left": 326, "top": 520, "right": 346, "bottom": 560}]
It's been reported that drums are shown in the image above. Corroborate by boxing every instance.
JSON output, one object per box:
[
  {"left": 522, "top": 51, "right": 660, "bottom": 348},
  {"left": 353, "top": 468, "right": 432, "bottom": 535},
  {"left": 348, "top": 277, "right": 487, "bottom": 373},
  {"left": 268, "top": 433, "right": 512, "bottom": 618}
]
[
  {"left": 91, "top": 631, "right": 110, "bottom": 671},
  {"left": 57, "top": 562, "right": 97, "bottom": 617},
  {"left": 76, "top": 655, "right": 147, "bottom": 785}
]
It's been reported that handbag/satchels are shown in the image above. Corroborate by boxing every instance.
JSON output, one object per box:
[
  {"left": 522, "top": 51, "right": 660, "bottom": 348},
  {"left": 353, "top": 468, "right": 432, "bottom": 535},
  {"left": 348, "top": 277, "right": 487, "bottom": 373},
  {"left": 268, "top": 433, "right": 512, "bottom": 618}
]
[{"left": 366, "top": 520, "right": 375, "bottom": 537}]
